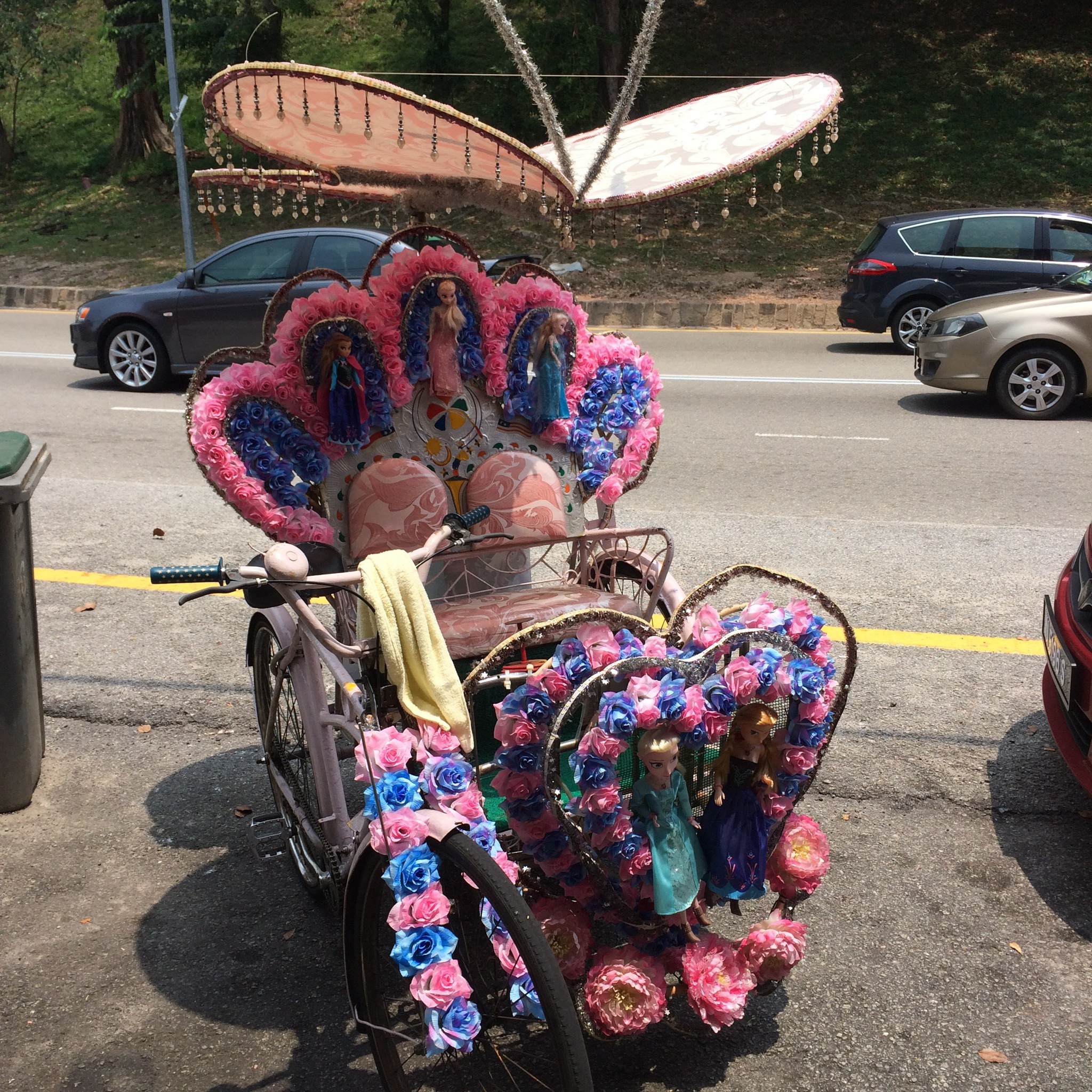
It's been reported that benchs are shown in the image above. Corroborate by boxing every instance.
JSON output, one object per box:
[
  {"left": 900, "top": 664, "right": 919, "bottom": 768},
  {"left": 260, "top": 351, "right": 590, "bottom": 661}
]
[{"left": 344, "top": 449, "right": 676, "bottom": 659}]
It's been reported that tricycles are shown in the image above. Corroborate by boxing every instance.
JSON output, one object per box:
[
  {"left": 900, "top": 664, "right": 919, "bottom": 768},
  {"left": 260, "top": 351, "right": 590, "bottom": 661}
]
[{"left": 179, "top": 0, "right": 862, "bottom": 1044}]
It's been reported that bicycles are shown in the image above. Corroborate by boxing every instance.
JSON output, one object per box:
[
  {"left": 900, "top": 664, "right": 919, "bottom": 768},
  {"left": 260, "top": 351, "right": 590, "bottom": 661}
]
[{"left": 150, "top": 504, "right": 595, "bottom": 1092}]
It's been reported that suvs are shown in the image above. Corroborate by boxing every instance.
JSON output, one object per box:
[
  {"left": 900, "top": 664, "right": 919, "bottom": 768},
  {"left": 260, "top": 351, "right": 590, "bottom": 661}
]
[{"left": 836, "top": 206, "right": 1092, "bottom": 356}]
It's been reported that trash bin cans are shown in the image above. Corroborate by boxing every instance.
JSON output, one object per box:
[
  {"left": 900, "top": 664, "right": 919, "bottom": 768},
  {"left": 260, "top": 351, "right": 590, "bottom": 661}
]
[{"left": 0, "top": 430, "right": 54, "bottom": 815}]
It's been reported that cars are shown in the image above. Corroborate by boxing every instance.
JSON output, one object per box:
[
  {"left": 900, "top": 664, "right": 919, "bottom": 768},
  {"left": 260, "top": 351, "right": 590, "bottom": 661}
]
[
  {"left": 913, "top": 261, "right": 1092, "bottom": 420},
  {"left": 1040, "top": 518, "right": 1092, "bottom": 801},
  {"left": 66, "top": 226, "right": 505, "bottom": 395}
]
